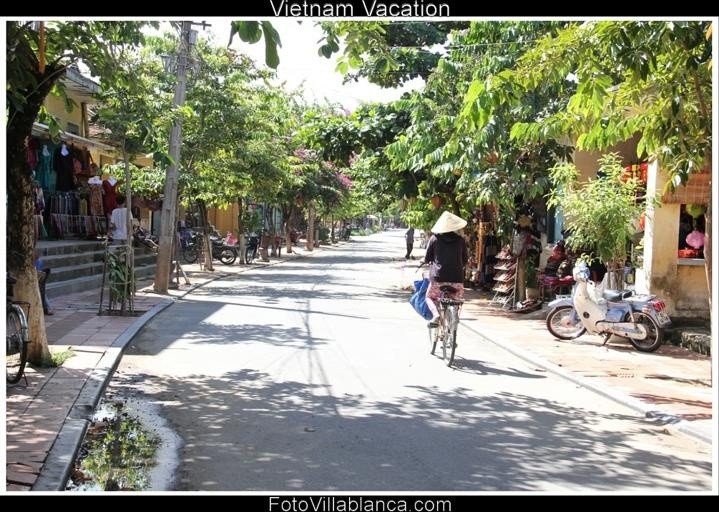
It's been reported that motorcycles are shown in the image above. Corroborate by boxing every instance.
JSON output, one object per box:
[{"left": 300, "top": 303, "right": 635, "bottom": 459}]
[
  {"left": 180, "top": 233, "right": 238, "bottom": 267},
  {"left": 545, "top": 258, "right": 675, "bottom": 354}
]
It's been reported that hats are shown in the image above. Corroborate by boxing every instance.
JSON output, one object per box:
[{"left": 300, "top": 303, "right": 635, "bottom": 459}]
[{"left": 430, "top": 210, "right": 468, "bottom": 234}]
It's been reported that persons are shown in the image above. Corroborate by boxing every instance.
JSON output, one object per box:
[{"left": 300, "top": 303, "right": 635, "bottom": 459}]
[
  {"left": 33, "top": 239, "right": 56, "bottom": 316},
  {"left": 404, "top": 224, "right": 415, "bottom": 259},
  {"left": 420, "top": 211, "right": 468, "bottom": 350},
  {"left": 108, "top": 196, "right": 134, "bottom": 266},
  {"left": 536, "top": 240, "right": 568, "bottom": 298}
]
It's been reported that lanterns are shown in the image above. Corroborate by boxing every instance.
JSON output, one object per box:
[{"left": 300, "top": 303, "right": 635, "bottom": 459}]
[
  {"left": 686, "top": 203, "right": 706, "bottom": 219},
  {"left": 686, "top": 230, "right": 706, "bottom": 250}
]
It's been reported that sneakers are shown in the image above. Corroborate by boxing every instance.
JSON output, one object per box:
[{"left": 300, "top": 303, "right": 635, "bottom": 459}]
[{"left": 427, "top": 318, "right": 439, "bottom": 329}]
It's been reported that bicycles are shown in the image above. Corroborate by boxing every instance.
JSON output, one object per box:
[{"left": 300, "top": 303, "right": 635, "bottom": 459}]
[
  {"left": 412, "top": 262, "right": 467, "bottom": 367},
  {"left": 6, "top": 272, "right": 35, "bottom": 391},
  {"left": 338, "top": 222, "right": 352, "bottom": 242},
  {"left": 245, "top": 230, "right": 261, "bottom": 264}
]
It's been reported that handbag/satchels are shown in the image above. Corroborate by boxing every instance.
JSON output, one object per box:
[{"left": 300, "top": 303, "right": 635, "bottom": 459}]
[{"left": 410, "top": 278, "right": 434, "bottom": 320}]
